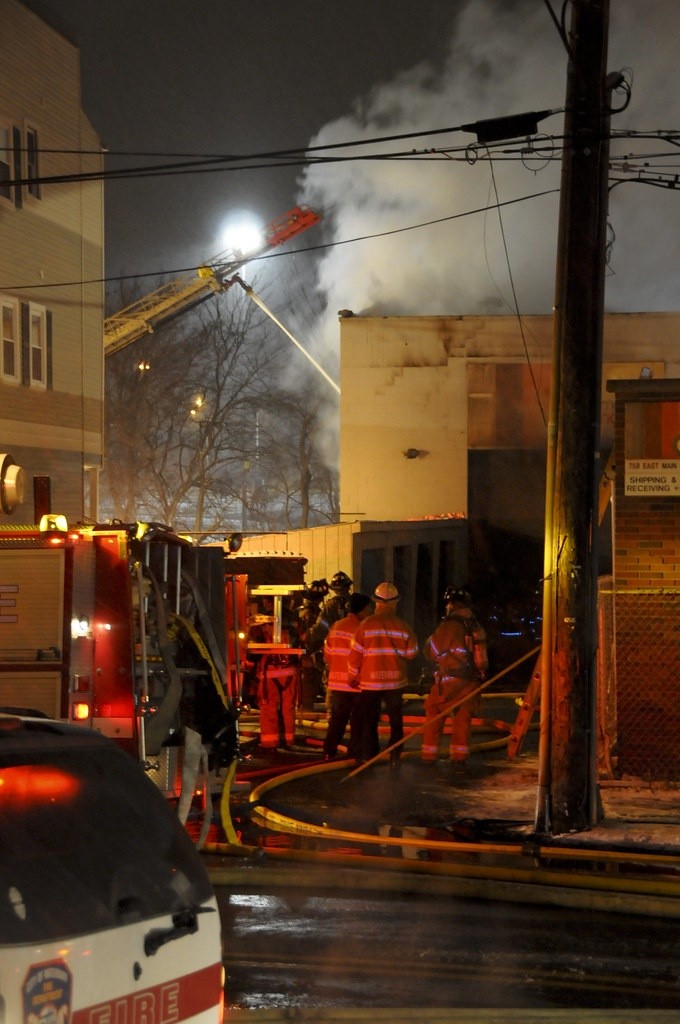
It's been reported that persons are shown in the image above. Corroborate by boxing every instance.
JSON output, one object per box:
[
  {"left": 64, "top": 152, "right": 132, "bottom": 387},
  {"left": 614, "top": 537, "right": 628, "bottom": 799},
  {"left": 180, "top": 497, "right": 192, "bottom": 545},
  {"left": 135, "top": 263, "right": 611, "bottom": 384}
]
[
  {"left": 348, "top": 581, "right": 418, "bottom": 770},
  {"left": 421, "top": 586, "right": 478, "bottom": 765},
  {"left": 255, "top": 571, "right": 373, "bottom": 760}
]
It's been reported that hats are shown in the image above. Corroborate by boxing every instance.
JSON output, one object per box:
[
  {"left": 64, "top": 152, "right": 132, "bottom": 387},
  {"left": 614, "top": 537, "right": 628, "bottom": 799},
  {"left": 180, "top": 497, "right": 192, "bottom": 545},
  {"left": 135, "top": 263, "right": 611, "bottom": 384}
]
[{"left": 349, "top": 592, "right": 372, "bottom": 613}]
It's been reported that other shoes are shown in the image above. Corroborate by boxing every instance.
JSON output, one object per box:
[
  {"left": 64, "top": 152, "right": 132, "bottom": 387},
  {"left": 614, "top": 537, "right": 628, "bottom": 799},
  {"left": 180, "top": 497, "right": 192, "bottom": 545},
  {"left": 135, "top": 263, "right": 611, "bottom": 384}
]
[
  {"left": 324, "top": 754, "right": 339, "bottom": 761},
  {"left": 451, "top": 760, "right": 464, "bottom": 777},
  {"left": 391, "top": 757, "right": 402, "bottom": 770}
]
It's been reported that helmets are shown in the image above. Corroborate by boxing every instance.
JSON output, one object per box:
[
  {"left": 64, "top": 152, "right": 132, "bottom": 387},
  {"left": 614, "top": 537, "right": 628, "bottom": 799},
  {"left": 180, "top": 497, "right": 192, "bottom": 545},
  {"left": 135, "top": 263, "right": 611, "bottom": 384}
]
[
  {"left": 374, "top": 582, "right": 401, "bottom": 604},
  {"left": 444, "top": 586, "right": 471, "bottom": 603},
  {"left": 329, "top": 572, "right": 353, "bottom": 591}
]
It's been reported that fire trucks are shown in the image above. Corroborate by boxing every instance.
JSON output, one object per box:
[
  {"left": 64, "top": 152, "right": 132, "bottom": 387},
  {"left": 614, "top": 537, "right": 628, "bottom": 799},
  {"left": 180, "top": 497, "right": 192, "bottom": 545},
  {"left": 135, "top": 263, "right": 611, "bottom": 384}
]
[{"left": 0, "top": 516, "right": 252, "bottom": 802}]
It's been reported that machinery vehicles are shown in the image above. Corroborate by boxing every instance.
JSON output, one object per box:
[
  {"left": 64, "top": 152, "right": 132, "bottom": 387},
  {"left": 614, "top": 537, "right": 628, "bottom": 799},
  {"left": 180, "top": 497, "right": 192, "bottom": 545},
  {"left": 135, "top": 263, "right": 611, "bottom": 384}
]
[{"left": 104, "top": 177, "right": 321, "bottom": 358}]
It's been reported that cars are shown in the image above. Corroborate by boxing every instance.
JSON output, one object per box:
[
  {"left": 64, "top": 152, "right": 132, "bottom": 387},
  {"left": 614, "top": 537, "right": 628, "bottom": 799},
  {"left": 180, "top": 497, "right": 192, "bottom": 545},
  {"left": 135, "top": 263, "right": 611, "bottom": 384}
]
[{"left": 0, "top": 714, "right": 226, "bottom": 1023}]
